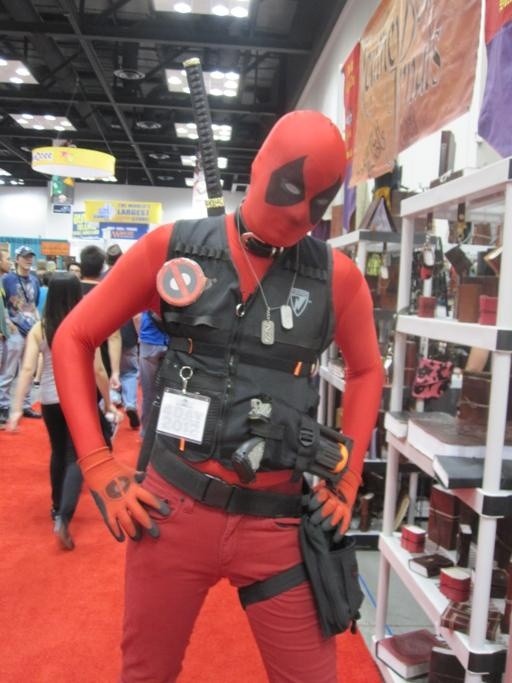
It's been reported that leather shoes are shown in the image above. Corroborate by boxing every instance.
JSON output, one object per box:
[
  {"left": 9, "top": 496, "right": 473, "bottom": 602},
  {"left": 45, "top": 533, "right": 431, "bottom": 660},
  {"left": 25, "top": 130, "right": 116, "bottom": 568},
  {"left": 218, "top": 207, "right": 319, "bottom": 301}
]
[{"left": 25, "top": 405, "right": 43, "bottom": 421}]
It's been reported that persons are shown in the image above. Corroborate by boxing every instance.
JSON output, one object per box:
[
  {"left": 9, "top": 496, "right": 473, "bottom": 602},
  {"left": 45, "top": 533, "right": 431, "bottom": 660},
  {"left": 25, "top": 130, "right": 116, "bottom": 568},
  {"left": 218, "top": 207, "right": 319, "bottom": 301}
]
[
  {"left": 100, "top": 246, "right": 140, "bottom": 429},
  {"left": 6, "top": 274, "right": 117, "bottom": 550},
  {"left": 1, "top": 245, "right": 41, "bottom": 419},
  {"left": 133, "top": 310, "right": 170, "bottom": 437},
  {"left": 77, "top": 247, "right": 122, "bottom": 404},
  {"left": 0, "top": 249, "right": 12, "bottom": 428},
  {"left": 52, "top": 109, "right": 384, "bottom": 683}
]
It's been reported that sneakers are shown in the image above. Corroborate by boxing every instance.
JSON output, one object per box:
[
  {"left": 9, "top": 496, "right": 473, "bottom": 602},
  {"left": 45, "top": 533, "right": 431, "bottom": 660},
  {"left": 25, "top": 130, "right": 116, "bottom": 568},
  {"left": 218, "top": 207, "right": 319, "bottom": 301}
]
[
  {"left": 41, "top": 504, "right": 76, "bottom": 552},
  {"left": 119, "top": 406, "right": 144, "bottom": 430}
]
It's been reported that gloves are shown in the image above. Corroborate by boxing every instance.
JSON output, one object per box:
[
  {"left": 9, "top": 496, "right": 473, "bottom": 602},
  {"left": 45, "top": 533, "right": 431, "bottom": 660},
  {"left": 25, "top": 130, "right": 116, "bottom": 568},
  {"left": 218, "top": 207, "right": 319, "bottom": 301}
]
[
  {"left": 304, "top": 459, "right": 371, "bottom": 539},
  {"left": 79, "top": 445, "right": 169, "bottom": 544}
]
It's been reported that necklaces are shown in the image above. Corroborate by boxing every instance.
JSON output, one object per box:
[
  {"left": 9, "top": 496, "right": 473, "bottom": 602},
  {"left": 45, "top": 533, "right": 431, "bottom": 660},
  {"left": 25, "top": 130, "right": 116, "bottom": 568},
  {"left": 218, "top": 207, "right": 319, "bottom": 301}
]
[
  {"left": 235, "top": 211, "right": 286, "bottom": 258},
  {"left": 237, "top": 204, "right": 299, "bottom": 346}
]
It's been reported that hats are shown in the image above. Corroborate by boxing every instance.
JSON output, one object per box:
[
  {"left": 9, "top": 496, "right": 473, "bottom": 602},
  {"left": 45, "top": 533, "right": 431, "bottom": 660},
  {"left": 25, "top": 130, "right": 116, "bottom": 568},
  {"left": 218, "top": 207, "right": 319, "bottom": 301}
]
[
  {"left": 14, "top": 243, "right": 36, "bottom": 260},
  {"left": 105, "top": 245, "right": 122, "bottom": 265}
]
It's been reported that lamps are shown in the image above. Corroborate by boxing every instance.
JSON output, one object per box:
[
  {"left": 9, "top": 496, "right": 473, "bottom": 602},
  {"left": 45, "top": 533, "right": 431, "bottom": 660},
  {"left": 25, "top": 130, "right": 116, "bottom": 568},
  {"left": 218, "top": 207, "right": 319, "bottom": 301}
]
[{"left": 31, "top": 146, "right": 116, "bottom": 179}]
